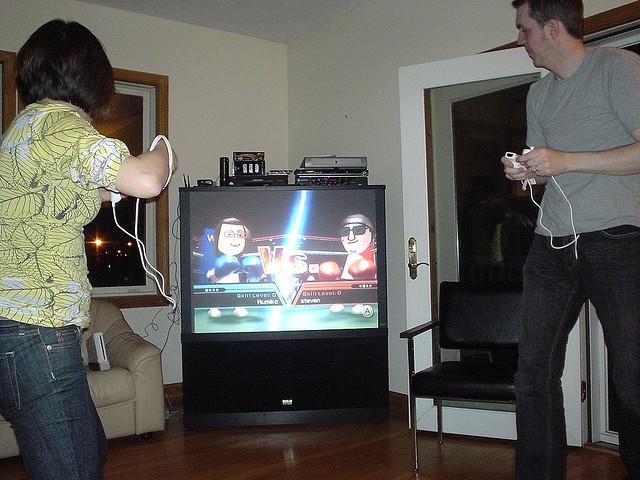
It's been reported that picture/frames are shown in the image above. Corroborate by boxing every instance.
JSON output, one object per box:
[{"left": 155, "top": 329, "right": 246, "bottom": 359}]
[
  {"left": 17, "top": 67, "right": 170, "bottom": 308},
  {"left": 0, "top": 50, "right": 17, "bottom": 140}
]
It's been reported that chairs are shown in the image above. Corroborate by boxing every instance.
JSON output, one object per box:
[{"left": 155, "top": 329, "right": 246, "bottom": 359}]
[{"left": 398, "top": 280, "right": 523, "bottom": 472}]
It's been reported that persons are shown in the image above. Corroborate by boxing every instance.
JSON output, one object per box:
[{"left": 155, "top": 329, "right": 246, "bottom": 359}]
[
  {"left": 319, "top": 214, "right": 378, "bottom": 315},
  {"left": 0, "top": 18, "right": 179, "bottom": 479},
  {"left": 206, "top": 217, "right": 262, "bottom": 316},
  {"left": 501, "top": 1, "right": 640, "bottom": 479}
]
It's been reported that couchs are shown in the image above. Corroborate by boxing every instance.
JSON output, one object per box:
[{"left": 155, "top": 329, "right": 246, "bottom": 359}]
[{"left": 0, "top": 296, "right": 167, "bottom": 460}]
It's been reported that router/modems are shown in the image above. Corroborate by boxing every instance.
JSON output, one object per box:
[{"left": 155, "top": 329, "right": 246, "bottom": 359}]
[{"left": 85, "top": 331, "right": 111, "bottom": 372}]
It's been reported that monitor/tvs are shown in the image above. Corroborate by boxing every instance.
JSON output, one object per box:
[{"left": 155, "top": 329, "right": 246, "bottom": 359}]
[{"left": 179, "top": 183, "right": 390, "bottom": 343}]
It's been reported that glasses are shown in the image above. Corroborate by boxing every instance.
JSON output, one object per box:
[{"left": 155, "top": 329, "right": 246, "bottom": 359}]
[
  {"left": 339, "top": 226, "right": 368, "bottom": 237},
  {"left": 223, "top": 232, "right": 248, "bottom": 238}
]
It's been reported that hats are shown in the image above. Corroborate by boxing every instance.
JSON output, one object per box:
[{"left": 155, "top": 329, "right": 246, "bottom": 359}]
[{"left": 340, "top": 214, "right": 375, "bottom": 236}]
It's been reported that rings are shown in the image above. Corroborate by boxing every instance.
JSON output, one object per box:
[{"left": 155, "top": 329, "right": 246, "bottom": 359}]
[{"left": 535, "top": 165, "right": 539, "bottom": 171}]
